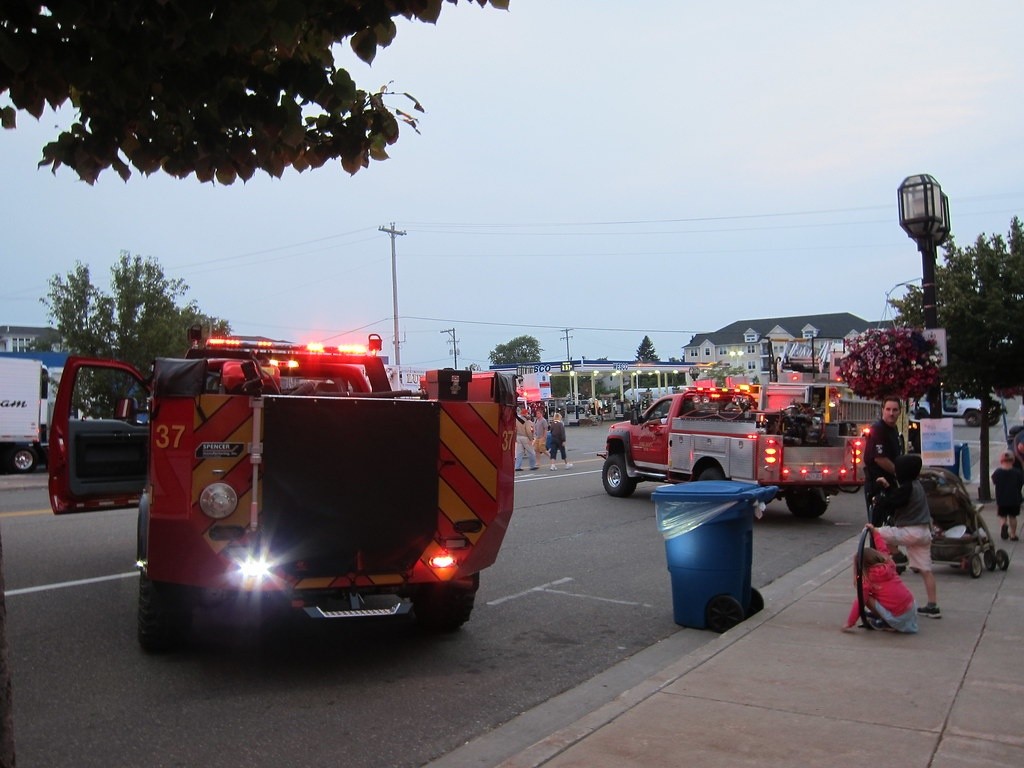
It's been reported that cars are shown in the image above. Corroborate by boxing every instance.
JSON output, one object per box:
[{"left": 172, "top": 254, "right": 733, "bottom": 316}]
[{"left": 909, "top": 383, "right": 1008, "bottom": 428}]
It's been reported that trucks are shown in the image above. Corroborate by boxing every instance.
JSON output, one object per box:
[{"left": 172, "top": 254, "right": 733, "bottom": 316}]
[{"left": 0, "top": 355, "right": 50, "bottom": 474}]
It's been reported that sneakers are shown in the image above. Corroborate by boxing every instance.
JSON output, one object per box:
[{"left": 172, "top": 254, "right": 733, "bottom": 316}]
[
  {"left": 917, "top": 606, "right": 942, "bottom": 618},
  {"left": 870, "top": 616, "right": 896, "bottom": 632}
]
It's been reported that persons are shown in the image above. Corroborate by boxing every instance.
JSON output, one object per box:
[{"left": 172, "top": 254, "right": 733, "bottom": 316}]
[
  {"left": 872, "top": 455, "right": 940, "bottom": 617},
  {"left": 839, "top": 525, "right": 919, "bottom": 632},
  {"left": 585, "top": 403, "right": 604, "bottom": 422},
  {"left": 864, "top": 396, "right": 906, "bottom": 575},
  {"left": 515, "top": 402, "right": 573, "bottom": 470},
  {"left": 991, "top": 450, "right": 1024, "bottom": 541}
]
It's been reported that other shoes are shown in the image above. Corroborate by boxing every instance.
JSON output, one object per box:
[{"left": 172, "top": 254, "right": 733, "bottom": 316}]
[
  {"left": 550, "top": 463, "right": 558, "bottom": 471},
  {"left": 1010, "top": 535, "right": 1019, "bottom": 541},
  {"left": 893, "top": 553, "right": 908, "bottom": 566},
  {"left": 564, "top": 462, "right": 574, "bottom": 469},
  {"left": 515, "top": 468, "right": 524, "bottom": 471},
  {"left": 531, "top": 467, "right": 539, "bottom": 471},
  {"left": 1001, "top": 524, "right": 1009, "bottom": 540}
]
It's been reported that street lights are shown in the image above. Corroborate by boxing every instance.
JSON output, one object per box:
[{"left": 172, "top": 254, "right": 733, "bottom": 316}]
[{"left": 895, "top": 173, "right": 951, "bottom": 419}]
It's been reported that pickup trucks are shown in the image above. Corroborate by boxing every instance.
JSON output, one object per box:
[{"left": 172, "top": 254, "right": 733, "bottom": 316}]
[{"left": 601, "top": 382, "right": 868, "bottom": 521}]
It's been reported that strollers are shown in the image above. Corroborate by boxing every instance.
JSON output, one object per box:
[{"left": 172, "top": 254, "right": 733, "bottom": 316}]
[{"left": 897, "top": 466, "right": 1010, "bottom": 579}]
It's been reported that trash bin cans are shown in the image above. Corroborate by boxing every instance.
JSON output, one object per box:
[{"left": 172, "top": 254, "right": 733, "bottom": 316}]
[
  {"left": 929, "top": 442, "right": 971, "bottom": 481},
  {"left": 650, "top": 480, "right": 779, "bottom": 633}
]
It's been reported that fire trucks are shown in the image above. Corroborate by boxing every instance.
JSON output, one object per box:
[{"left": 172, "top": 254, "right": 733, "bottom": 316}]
[{"left": 43, "top": 324, "right": 522, "bottom": 660}]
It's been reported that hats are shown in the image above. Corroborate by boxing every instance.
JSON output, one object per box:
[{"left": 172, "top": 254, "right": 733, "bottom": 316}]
[
  {"left": 521, "top": 409, "right": 529, "bottom": 414},
  {"left": 1000, "top": 450, "right": 1015, "bottom": 462}
]
